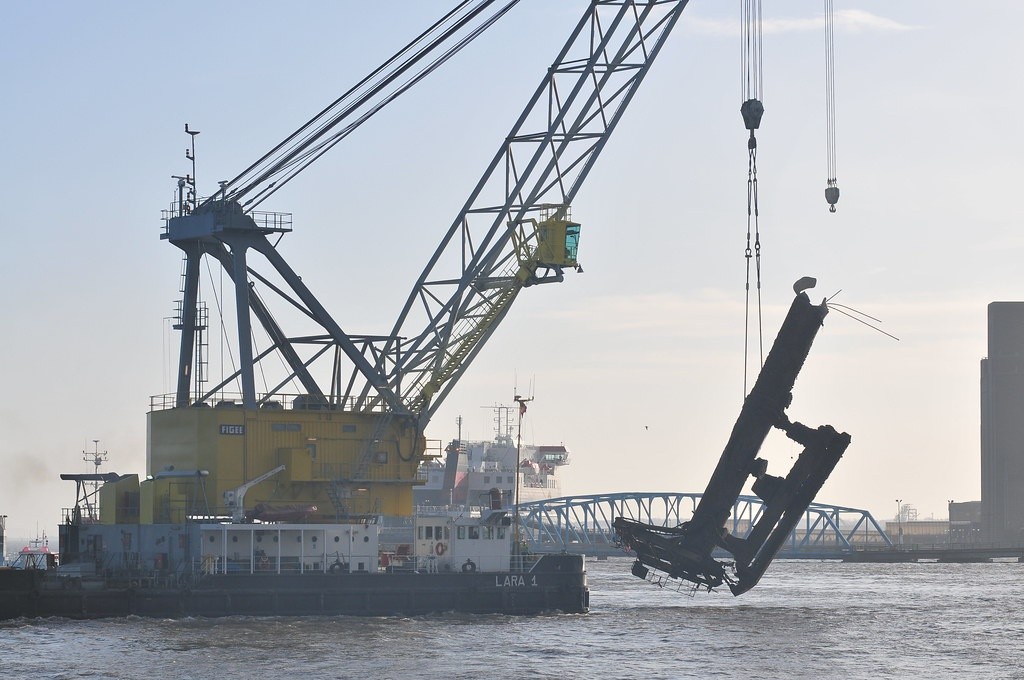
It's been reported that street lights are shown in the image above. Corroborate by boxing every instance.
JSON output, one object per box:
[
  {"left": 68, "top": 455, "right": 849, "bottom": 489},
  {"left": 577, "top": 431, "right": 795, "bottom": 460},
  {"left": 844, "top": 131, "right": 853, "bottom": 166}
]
[
  {"left": 896, "top": 499, "right": 902, "bottom": 546},
  {"left": 948, "top": 500, "right": 953, "bottom": 546}
]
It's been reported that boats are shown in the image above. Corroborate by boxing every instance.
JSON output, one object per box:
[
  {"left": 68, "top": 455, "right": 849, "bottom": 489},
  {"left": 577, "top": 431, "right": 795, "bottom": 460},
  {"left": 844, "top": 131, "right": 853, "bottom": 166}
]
[{"left": 0, "top": 0, "right": 854, "bottom": 620}]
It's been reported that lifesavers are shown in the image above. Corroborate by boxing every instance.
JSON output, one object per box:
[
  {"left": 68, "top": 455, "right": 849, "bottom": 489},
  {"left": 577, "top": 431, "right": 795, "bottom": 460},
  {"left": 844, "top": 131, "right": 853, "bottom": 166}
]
[
  {"left": 436, "top": 542, "right": 444, "bottom": 555},
  {"left": 462, "top": 562, "right": 476, "bottom": 573},
  {"left": 331, "top": 562, "right": 344, "bottom": 574}
]
